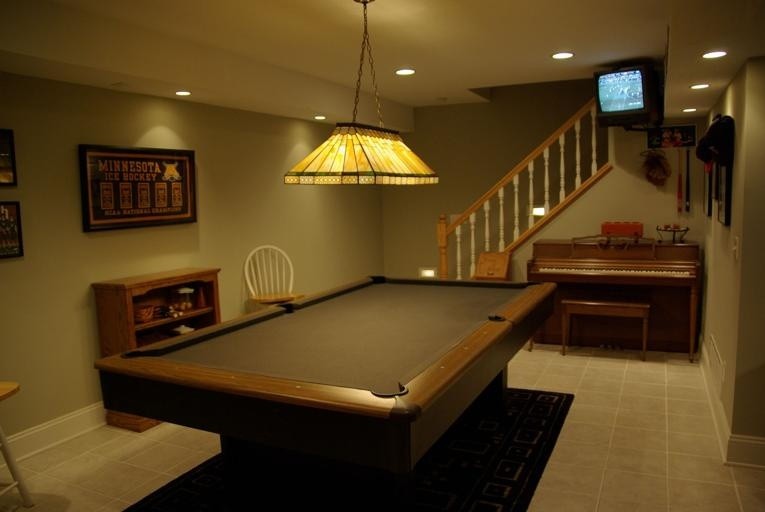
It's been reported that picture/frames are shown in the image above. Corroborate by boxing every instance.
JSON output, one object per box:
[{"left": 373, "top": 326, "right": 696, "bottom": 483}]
[
  {"left": 77, "top": 143, "right": 197, "bottom": 232},
  {"left": 0, "top": 201, "right": 23, "bottom": 259},
  {"left": 703, "top": 112, "right": 734, "bottom": 229},
  {"left": 0, "top": 129, "right": 18, "bottom": 188},
  {"left": 647, "top": 125, "right": 698, "bottom": 149}
]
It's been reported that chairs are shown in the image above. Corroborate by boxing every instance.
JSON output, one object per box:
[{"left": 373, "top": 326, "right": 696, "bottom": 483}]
[
  {"left": 243, "top": 244, "right": 305, "bottom": 304},
  {"left": 472, "top": 249, "right": 513, "bottom": 282}
]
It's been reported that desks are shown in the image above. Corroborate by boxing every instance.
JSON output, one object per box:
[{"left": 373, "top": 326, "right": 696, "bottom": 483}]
[
  {"left": 247, "top": 291, "right": 305, "bottom": 305},
  {"left": 0, "top": 379, "right": 35, "bottom": 511},
  {"left": 92, "top": 277, "right": 560, "bottom": 511}
]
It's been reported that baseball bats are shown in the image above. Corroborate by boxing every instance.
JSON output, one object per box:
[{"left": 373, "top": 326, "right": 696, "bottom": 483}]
[
  {"left": 678, "top": 149, "right": 683, "bottom": 213},
  {"left": 686, "top": 149, "right": 690, "bottom": 212}
]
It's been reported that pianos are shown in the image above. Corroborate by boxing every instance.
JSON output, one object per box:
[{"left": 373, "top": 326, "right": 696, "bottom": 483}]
[{"left": 526, "top": 235, "right": 700, "bottom": 363}]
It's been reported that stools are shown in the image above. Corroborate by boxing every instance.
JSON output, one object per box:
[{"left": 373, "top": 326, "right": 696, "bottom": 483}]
[{"left": 560, "top": 295, "right": 651, "bottom": 362}]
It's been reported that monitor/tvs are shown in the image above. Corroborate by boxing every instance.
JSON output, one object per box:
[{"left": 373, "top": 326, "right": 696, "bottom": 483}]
[{"left": 593, "top": 64, "right": 657, "bottom": 117}]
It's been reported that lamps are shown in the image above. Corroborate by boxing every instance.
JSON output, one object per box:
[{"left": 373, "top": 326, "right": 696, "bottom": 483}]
[{"left": 284, "top": 0, "right": 440, "bottom": 187}]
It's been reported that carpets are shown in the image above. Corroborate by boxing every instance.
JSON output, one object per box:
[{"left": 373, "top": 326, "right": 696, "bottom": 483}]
[{"left": 122, "top": 386, "right": 576, "bottom": 512}]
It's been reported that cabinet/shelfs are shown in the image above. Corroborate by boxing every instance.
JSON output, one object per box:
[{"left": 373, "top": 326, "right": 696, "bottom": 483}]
[{"left": 89, "top": 267, "right": 222, "bottom": 434}]
